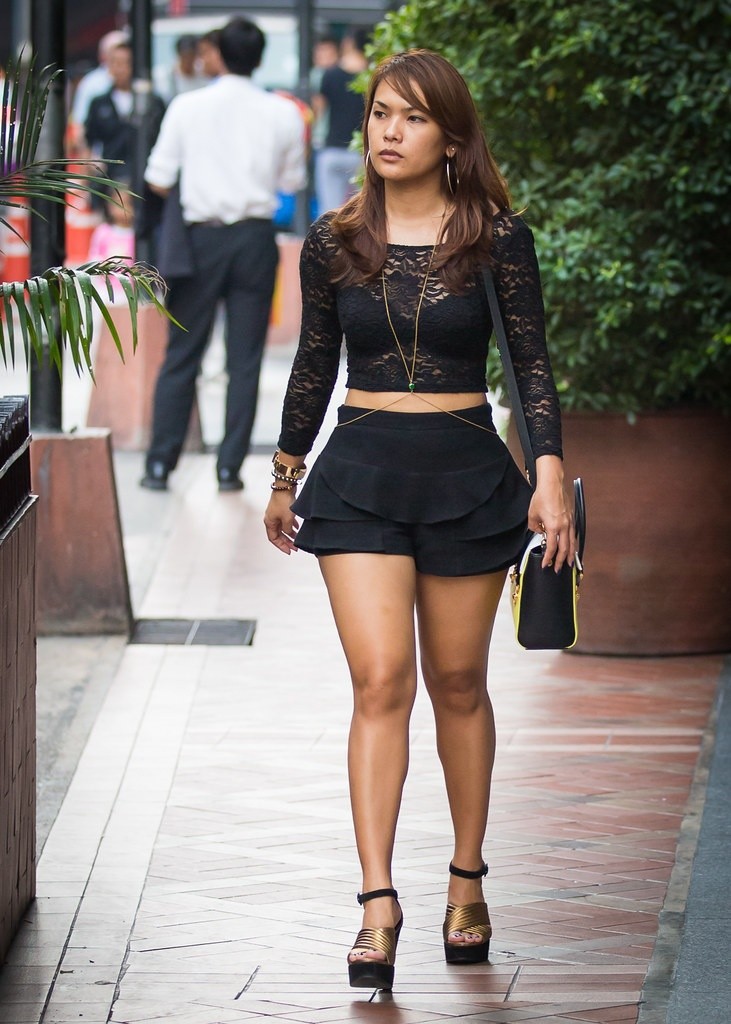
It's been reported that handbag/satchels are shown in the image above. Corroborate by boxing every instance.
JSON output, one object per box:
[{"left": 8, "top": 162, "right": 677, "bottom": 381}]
[{"left": 509, "top": 478, "right": 586, "bottom": 650}]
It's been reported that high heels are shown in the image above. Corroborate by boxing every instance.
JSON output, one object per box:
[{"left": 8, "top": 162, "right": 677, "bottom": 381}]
[
  {"left": 443, "top": 860, "right": 492, "bottom": 963},
  {"left": 347, "top": 888, "right": 403, "bottom": 989}
]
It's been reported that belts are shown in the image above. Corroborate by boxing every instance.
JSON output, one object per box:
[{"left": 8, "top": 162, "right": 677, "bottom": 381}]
[{"left": 190, "top": 219, "right": 272, "bottom": 228}]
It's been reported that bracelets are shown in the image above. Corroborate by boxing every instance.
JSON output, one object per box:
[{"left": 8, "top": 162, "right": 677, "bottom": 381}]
[{"left": 270, "top": 450, "right": 307, "bottom": 490}]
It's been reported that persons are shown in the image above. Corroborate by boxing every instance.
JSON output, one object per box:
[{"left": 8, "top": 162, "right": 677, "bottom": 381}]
[
  {"left": 141, "top": 17, "right": 307, "bottom": 493},
  {"left": 69, "top": 29, "right": 371, "bottom": 302},
  {"left": 261, "top": 50, "right": 586, "bottom": 991}
]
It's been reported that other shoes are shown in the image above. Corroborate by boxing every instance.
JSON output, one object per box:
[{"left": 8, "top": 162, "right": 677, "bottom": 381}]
[
  {"left": 140, "top": 462, "right": 168, "bottom": 489},
  {"left": 218, "top": 470, "right": 243, "bottom": 490}
]
